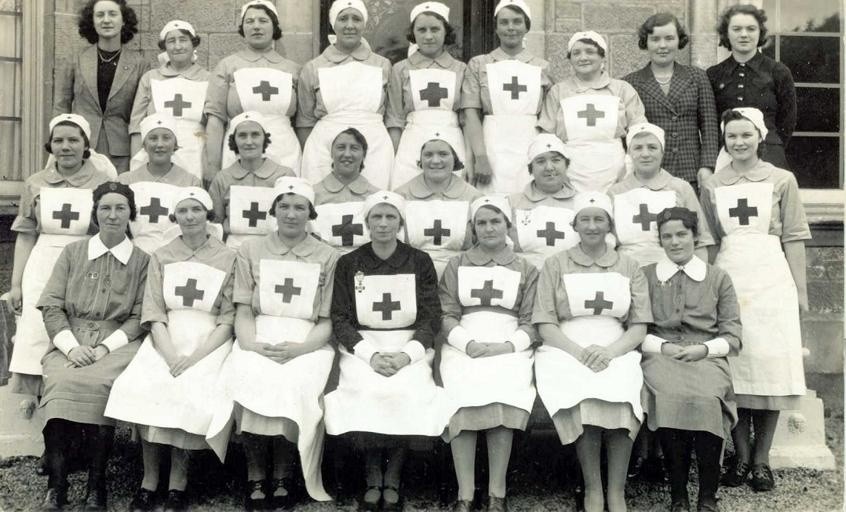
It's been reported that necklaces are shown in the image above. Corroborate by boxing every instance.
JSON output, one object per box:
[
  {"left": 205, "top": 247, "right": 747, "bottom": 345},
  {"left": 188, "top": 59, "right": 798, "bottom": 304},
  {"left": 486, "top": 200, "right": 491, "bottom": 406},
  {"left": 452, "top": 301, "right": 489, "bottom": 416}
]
[{"left": 98, "top": 49, "right": 120, "bottom": 63}]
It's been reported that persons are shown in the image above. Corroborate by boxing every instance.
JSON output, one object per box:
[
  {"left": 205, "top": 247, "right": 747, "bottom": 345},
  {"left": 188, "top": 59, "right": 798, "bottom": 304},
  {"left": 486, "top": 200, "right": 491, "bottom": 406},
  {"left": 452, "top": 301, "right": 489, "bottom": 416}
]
[
  {"left": 329, "top": 190, "right": 442, "bottom": 512},
  {"left": 633, "top": 207, "right": 743, "bottom": 512},
  {"left": 530, "top": 191, "right": 655, "bottom": 512},
  {"left": 53, "top": 0, "right": 151, "bottom": 175},
  {"left": 437, "top": 194, "right": 539, "bottom": 512},
  {"left": 115, "top": 0, "right": 718, "bottom": 264},
  {"left": 699, "top": 106, "right": 813, "bottom": 492},
  {"left": 231, "top": 176, "right": 341, "bottom": 512},
  {"left": 8, "top": 113, "right": 111, "bottom": 420},
  {"left": 35, "top": 181, "right": 151, "bottom": 512},
  {"left": 705, "top": 4, "right": 797, "bottom": 170},
  {"left": 102, "top": 186, "right": 235, "bottom": 512}
]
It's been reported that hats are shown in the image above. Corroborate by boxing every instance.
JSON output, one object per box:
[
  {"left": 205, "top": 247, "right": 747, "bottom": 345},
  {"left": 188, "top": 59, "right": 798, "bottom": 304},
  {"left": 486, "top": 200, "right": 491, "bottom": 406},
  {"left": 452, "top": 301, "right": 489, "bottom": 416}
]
[
  {"left": 363, "top": 191, "right": 405, "bottom": 227},
  {"left": 495, "top": 1, "right": 532, "bottom": 21},
  {"left": 657, "top": 207, "right": 696, "bottom": 231},
  {"left": 627, "top": 122, "right": 668, "bottom": 150},
  {"left": 49, "top": 113, "right": 91, "bottom": 139},
  {"left": 269, "top": 176, "right": 316, "bottom": 218},
  {"left": 471, "top": 195, "right": 513, "bottom": 226},
  {"left": 170, "top": 186, "right": 214, "bottom": 219},
  {"left": 414, "top": 130, "right": 464, "bottom": 170},
  {"left": 326, "top": 124, "right": 369, "bottom": 156},
  {"left": 720, "top": 106, "right": 768, "bottom": 142},
  {"left": 241, "top": 1, "right": 279, "bottom": 21},
  {"left": 159, "top": 20, "right": 195, "bottom": 40},
  {"left": 566, "top": 30, "right": 606, "bottom": 55},
  {"left": 408, "top": 0, "right": 452, "bottom": 26},
  {"left": 571, "top": 191, "right": 613, "bottom": 228},
  {"left": 527, "top": 133, "right": 571, "bottom": 166},
  {"left": 328, "top": 1, "right": 368, "bottom": 29},
  {"left": 229, "top": 111, "right": 271, "bottom": 137},
  {"left": 138, "top": 113, "right": 185, "bottom": 147},
  {"left": 93, "top": 181, "right": 134, "bottom": 203}
]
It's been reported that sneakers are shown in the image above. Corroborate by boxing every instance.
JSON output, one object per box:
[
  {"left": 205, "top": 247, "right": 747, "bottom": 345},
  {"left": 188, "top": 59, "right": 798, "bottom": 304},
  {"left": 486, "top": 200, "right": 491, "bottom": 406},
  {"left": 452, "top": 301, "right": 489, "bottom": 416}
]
[
  {"left": 628, "top": 456, "right": 647, "bottom": 477},
  {"left": 671, "top": 499, "right": 720, "bottom": 511},
  {"left": 84, "top": 490, "right": 104, "bottom": 512},
  {"left": 652, "top": 454, "right": 665, "bottom": 467},
  {"left": 489, "top": 495, "right": 505, "bottom": 512},
  {"left": 455, "top": 500, "right": 474, "bottom": 512},
  {"left": 129, "top": 486, "right": 154, "bottom": 512},
  {"left": 163, "top": 489, "right": 185, "bottom": 511},
  {"left": 41, "top": 488, "right": 60, "bottom": 511},
  {"left": 723, "top": 460, "right": 774, "bottom": 491}
]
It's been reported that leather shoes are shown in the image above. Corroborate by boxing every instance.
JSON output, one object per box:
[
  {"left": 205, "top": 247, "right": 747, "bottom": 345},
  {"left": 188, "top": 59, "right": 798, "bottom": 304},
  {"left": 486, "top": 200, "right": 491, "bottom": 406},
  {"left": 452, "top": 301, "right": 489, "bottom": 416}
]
[
  {"left": 36, "top": 461, "right": 45, "bottom": 474},
  {"left": 358, "top": 486, "right": 403, "bottom": 510},
  {"left": 246, "top": 476, "right": 292, "bottom": 511}
]
